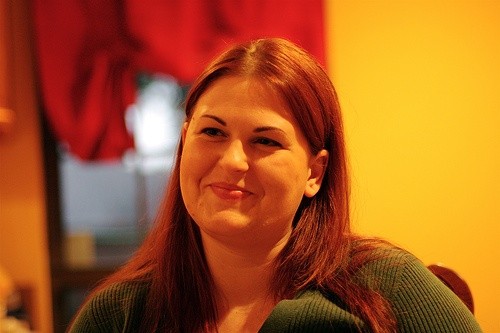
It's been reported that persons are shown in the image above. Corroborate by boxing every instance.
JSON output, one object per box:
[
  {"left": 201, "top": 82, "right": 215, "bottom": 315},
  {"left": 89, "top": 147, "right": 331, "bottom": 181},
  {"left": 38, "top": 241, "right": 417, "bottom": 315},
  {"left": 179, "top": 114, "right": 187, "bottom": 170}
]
[{"left": 60, "top": 37, "right": 484, "bottom": 333}]
